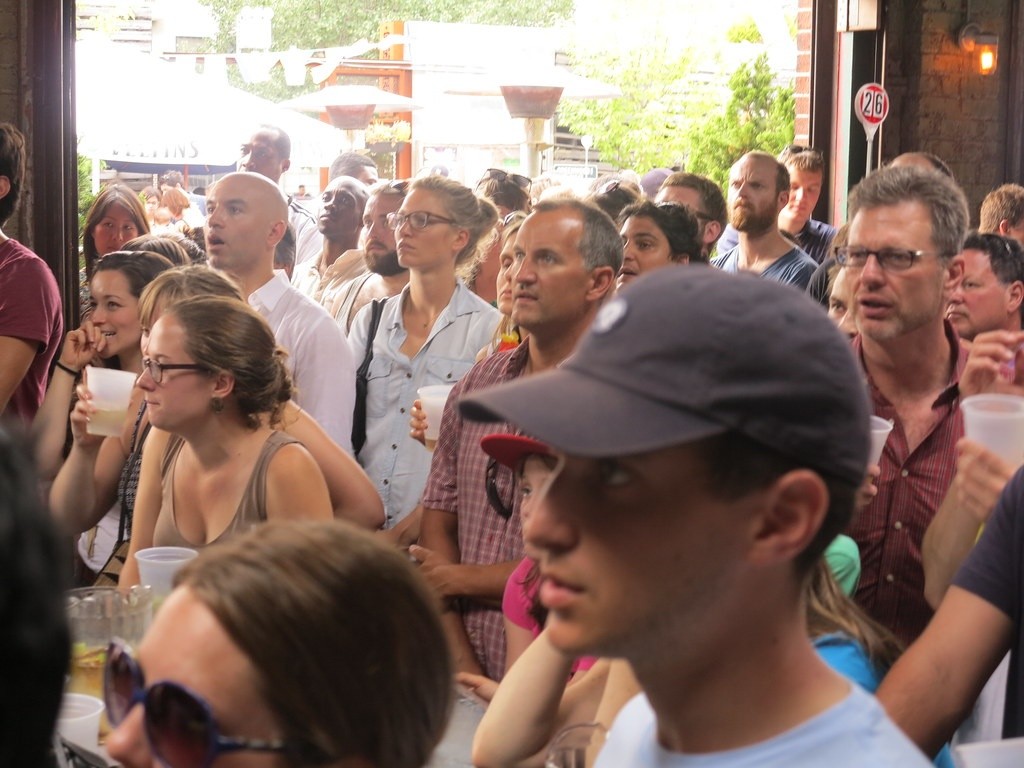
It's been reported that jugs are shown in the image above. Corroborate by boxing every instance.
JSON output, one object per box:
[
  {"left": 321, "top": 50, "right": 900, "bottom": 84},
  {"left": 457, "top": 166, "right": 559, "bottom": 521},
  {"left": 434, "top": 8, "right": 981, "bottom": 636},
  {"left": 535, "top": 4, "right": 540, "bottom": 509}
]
[{"left": 65, "top": 584, "right": 155, "bottom": 747}]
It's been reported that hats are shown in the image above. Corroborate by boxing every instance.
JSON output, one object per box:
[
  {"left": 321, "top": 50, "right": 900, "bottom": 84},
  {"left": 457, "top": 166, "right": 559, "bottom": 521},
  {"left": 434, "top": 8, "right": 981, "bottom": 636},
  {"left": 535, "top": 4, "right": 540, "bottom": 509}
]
[
  {"left": 456, "top": 266, "right": 871, "bottom": 484},
  {"left": 640, "top": 167, "right": 674, "bottom": 201},
  {"left": 480, "top": 434, "right": 551, "bottom": 475}
]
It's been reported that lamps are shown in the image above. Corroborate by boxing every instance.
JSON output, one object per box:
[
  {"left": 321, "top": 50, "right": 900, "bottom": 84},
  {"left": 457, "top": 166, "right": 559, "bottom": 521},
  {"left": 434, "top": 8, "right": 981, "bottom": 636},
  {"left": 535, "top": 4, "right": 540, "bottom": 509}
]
[{"left": 958, "top": 22, "right": 999, "bottom": 75}]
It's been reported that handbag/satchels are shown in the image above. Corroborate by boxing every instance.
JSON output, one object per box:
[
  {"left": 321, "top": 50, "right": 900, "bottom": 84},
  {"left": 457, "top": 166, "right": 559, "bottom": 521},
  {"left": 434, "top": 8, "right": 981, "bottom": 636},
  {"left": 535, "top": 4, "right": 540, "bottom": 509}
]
[{"left": 91, "top": 540, "right": 129, "bottom": 587}]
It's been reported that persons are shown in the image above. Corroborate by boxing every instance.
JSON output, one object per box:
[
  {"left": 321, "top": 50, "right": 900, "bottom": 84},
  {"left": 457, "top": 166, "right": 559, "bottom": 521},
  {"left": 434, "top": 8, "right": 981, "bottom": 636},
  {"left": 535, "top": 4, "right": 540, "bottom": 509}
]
[{"left": 0, "top": 122, "right": 1024, "bottom": 768}]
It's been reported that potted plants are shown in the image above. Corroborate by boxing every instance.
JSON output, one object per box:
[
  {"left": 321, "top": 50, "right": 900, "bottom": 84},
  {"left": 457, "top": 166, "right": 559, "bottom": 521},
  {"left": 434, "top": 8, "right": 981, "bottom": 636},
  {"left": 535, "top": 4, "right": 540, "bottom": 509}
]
[{"left": 366, "top": 123, "right": 411, "bottom": 153}]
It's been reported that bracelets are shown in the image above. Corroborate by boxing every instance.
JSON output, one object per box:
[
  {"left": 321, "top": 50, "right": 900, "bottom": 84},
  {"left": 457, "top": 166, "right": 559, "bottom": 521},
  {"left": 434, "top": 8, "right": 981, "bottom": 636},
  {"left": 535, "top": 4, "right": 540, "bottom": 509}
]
[{"left": 56, "top": 361, "right": 79, "bottom": 376}]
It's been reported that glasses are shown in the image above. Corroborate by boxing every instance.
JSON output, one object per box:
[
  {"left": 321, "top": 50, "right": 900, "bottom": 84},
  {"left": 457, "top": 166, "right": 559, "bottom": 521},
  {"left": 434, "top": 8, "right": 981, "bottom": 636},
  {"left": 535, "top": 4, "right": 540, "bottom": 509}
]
[
  {"left": 475, "top": 169, "right": 533, "bottom": 197},
  {"left": 834, "top": 246, "right": 942, "bottom": 270},
  {"left": 482, "top": 458, "right": 517, "bottom": 517},
  {"left": 502, "top": 211, "right": 522, "bottom": 227},
  {"left": 780, "top": 144, "right": 824, "bottom": 159},
  {"left": 386, "top": 211, "right": 453, "bottom": 230},
  {"left": 597, "top": 180, "right": 630, "bottom": 204},
  {"left": 102, "top": 639, "right": 312, "bottom": 768},
  {"left": 141, "top": 356, "right": 204, "bottom": 384},
  {"left": 388, "top": 179, "right": 407, "bottom": 201}
]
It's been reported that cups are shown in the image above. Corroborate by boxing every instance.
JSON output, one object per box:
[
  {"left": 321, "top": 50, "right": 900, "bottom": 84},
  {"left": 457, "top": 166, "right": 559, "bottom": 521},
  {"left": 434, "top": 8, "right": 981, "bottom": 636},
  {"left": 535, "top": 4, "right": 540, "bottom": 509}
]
[
  {"left": 54, "top": 693, "right": 106, "bottom": 768},
  {"left": 134, "top": 547, "right": 199, "bottom": 610},
  {"left": 417, "top": 383, "right": 453, "bottom": 453},
  {"left": 86, "top": 365, "right": 137, "bottom": 439},
  {"left": 959, "top": 393, "right": 1024, "bottom": 469},
  {"left": 864, "top": 416, "right": 894, "bottom": 483}
]
